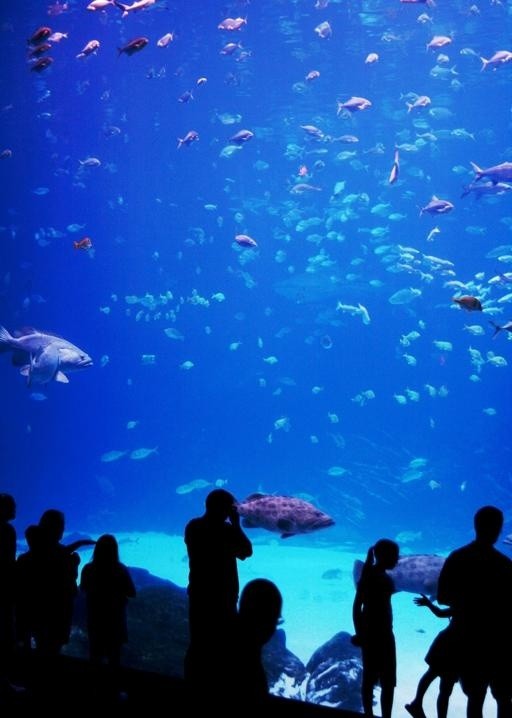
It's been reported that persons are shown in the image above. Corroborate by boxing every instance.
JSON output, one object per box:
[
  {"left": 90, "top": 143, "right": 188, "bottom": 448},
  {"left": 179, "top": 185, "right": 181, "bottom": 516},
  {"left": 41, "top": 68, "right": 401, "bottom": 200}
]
[
  {"left": 349, "top": 540, "right": 409, "bottom": 718},
  {"left": 184, "top": 578, "right": 281, "bottom": 718},
  {"left": 184, "top": 490, "right": 252, "bottom": 660},
  {"left": 1, "top": 493, "right": 138, "bottom": 718},
  {"left": 405, "top": 593, "right": 457, "bottom": 718},
  {"left": 438, "top": 506, "right": 512, "bottom": 718}
]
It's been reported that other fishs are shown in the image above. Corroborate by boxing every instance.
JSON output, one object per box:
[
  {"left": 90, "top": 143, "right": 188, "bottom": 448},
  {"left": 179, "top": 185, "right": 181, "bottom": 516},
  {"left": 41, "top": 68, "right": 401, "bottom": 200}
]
[{"left": 0, "top": 0, "right": 511, "bottom": 604}]
